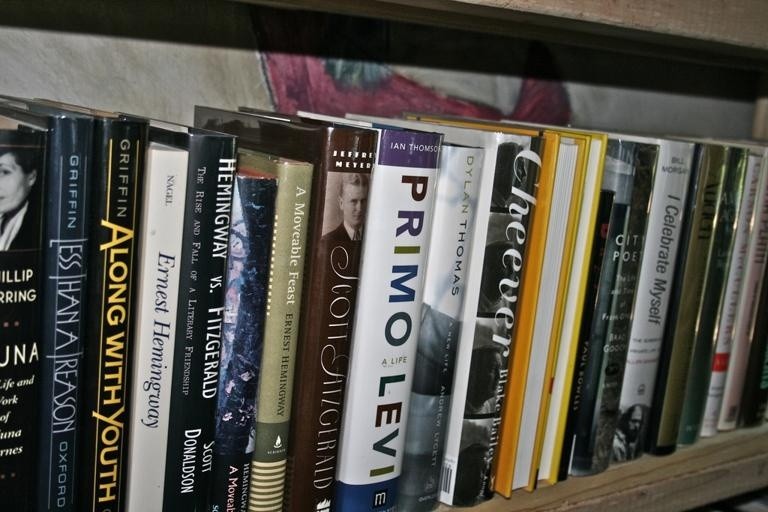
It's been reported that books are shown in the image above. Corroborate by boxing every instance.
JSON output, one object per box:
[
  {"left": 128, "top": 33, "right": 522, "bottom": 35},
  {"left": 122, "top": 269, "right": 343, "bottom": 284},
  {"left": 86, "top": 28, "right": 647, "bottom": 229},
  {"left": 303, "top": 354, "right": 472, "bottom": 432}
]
[{"left": 1, "top": 95, "right": 768, "bottom": 511}]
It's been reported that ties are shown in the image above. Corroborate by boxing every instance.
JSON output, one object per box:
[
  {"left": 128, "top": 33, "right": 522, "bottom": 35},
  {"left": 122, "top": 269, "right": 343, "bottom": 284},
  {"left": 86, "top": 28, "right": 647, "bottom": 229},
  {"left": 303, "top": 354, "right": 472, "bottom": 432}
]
[{"left": 352, "top": 230, "right": 362, "bottom": 242}]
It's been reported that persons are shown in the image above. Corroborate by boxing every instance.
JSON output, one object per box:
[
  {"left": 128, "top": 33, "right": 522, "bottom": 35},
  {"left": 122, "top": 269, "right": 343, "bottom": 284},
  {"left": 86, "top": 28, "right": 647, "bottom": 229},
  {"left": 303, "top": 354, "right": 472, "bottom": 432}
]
[
  {"left": 323, "top": 174, "right": 367, "bottom": 240},
  {"left": 476, "top": 243, "right": 515, "bottom": 312},
  {"left": 2, "top": 147, "right": 40, "bottom": 249},
  {"left": 489, "top": 143, "right": 527, "bottom": 206},
  {"left": 613, "top": 404, "right": 643, "bottom": 463},
  {"left": 467, "top": 346, "right": 503, "bottom": 414}
]
